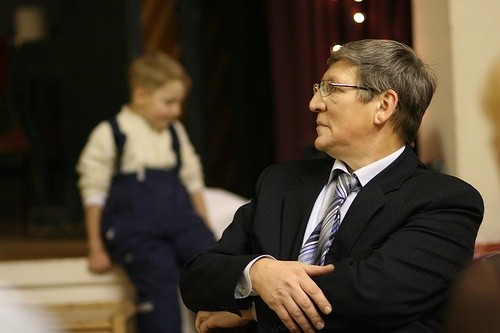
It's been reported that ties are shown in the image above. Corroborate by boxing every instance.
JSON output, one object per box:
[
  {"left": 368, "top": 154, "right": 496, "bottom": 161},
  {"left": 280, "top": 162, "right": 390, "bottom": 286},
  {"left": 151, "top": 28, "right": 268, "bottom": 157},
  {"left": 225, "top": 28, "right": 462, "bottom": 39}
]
[{"left": 298, "top": 172, "right": 358, "bottom": 264}]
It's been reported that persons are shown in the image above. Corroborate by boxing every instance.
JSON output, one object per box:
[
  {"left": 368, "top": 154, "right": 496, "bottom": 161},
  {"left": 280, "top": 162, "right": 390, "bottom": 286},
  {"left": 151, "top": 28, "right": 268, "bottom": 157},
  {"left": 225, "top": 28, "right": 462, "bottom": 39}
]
[
  {"left": 76, "top": 52, "right": 211, "bottom": 333},
  {"left": 177, "top": 39, "right": 485, "bottom": 333}
]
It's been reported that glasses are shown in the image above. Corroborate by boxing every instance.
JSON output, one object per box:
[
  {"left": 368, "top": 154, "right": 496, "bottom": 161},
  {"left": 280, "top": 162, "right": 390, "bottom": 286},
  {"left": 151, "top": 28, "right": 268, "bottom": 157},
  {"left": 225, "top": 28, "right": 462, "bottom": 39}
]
[{"left": 313, "top": 80, "right": 378, "bottom": 97}]
